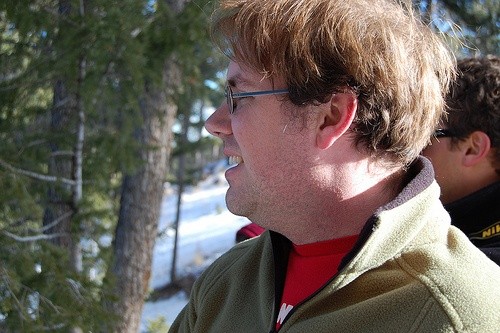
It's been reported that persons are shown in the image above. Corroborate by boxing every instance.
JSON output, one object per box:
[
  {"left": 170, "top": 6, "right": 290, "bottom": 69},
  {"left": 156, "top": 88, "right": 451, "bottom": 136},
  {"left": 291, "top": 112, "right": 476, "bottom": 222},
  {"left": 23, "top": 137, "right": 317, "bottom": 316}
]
[
  {"left": 164, "top": 1, "right": 500, "bottom": 333},
  {"left": 419, "top": 52, "right": 500, "bottom": 267}
]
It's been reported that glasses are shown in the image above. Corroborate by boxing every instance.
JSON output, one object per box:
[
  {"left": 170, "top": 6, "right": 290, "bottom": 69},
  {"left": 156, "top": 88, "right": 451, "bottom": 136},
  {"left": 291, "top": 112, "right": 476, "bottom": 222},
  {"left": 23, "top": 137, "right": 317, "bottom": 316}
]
[
  {"left": 223, "top": 83, "right": 289, "bottom": 115},
  {"left": 433, "top": 125, "right": 497, "bottom": 149}
]
